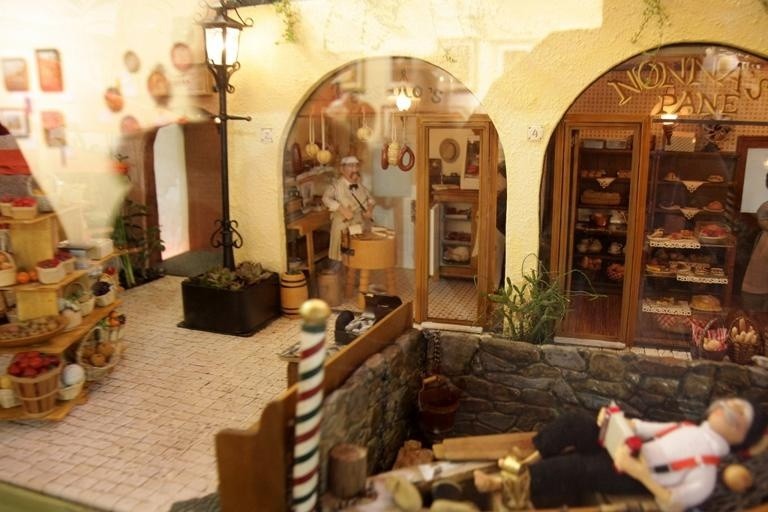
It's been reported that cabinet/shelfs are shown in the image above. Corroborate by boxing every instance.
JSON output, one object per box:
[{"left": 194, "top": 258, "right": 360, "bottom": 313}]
[
  {"left": 627, "top": 114, "right": 741, "bottom": 348},
  {"left": 428, "top": 187, "right": 482, "bottom": 281},
  {"left": 556, "top": 115, "right": 644, "bottom": 350},
  {"left": 0, "top": 204, "right": 131, "bottom": 422}
]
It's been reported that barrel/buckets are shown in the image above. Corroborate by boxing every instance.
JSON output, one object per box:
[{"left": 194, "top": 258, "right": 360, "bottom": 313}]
[{"left": 279, "top": 269, "right": 308, "bottom": 319}]
